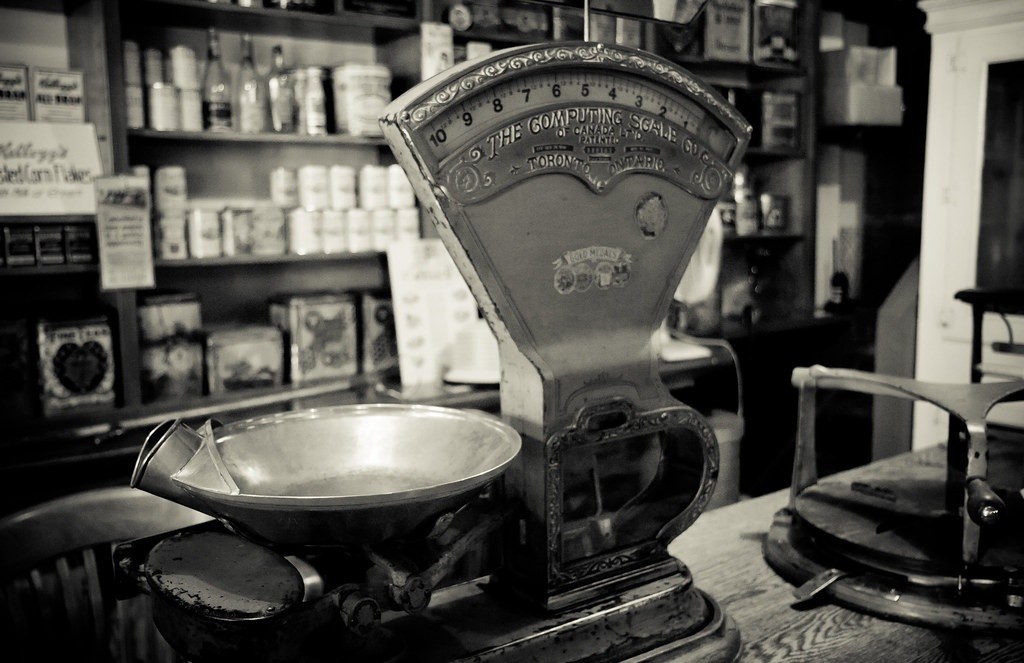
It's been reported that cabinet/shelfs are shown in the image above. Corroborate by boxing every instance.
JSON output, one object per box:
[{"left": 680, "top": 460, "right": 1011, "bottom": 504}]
[{"left": 0, "top": 0, "right": 933, "bottom": 500}]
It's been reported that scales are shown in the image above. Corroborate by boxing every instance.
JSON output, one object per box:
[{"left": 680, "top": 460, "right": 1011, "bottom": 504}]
[{"left": 110, "top": 38, "right": 757, "bottom": 663}]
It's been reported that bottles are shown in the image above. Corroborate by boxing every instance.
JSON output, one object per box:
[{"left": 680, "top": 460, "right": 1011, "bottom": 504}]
[
  {"left": 305, "top": 67, "right": 328, "bottom": 135},
  {"left": 236, "top": 31, "right": 268, "bottom": 134},
  {"left": 202, "top": 28, "right": 233, "bottom": 133},
  {"left": 266, "top": 45, "right": 294, "bottom": 134}
]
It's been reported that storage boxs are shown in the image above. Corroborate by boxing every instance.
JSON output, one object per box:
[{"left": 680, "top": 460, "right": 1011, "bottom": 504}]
[
  {"left": 819, "top": 11, "right": 903, "bottom": 125},
  {"left": 141, "top": 291, "right": 396, "bottom": 402}
]
